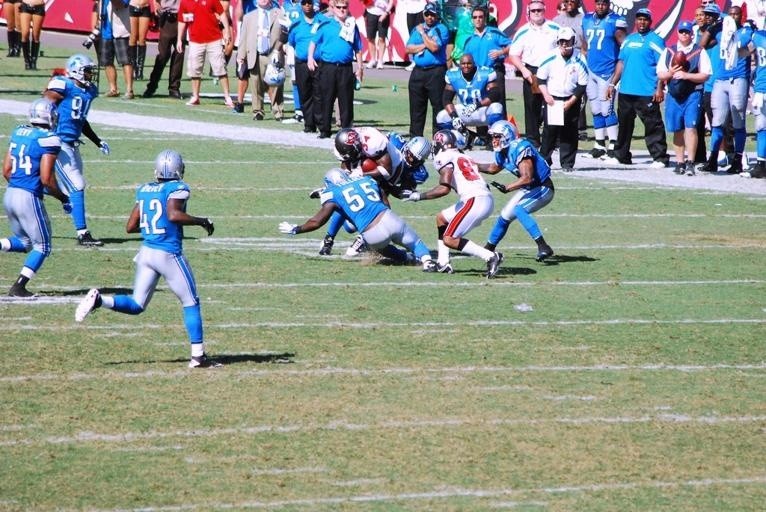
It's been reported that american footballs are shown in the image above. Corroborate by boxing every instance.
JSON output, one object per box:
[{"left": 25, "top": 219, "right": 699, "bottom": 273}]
[
  {"left": 672, "top": 51, "right": 686, "bottom": 69},
  {"left": 361, "top": 159, "right": 377, "bottom": 172}
]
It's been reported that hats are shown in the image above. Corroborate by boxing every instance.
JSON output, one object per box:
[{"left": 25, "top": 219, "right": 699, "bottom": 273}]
[
  {"left": 556, "top": 4, "right": 720, "bottom": 41},
  {"left": 422, "top": 2, "right": 436, "bottom": 14}
]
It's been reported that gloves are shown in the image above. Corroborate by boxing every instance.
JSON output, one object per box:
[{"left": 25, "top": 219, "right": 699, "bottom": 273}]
[
  {"left": 200, "top": 218, "right": 213, "bottom": 235},
  {"left": 272, "top": 49, "right": 279, "bottom": 65},
  {"left": 398, "top": 187, "right": 420, "bottom": 202},
  {"left": 62, "top": 199, "right": 72, "bottom": 214},
  {"left": 490, "top": 182, "right": 506, "bottom": 194},
  {"left": 278, "top": 221, "right": 297, "bottom": 234},
  {"left": 98, "top": 140, "right": 110, "bottom": 155}
]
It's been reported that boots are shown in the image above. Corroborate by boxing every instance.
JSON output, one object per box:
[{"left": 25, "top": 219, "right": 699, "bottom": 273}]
[{"left": 6, "top": 30, "right": 145, "bottom": 80}]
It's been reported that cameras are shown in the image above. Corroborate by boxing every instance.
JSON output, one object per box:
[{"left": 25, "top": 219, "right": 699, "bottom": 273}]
[{"left": 82, "top": 23, "right": 101, "bottom": 49}]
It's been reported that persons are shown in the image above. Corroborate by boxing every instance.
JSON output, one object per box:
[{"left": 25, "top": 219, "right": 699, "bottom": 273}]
[
  {"left": 405, "top": 2, "right": 450, "bottom": 139},
  {"left": 2, "top": 2, "right": 22, "bottom": 57},
  {"left": 42, "top": 54, "right": 109, "bottom": 247},
  {"left": 279, "top": 166, "right": 437, "bottom": 273},
  {"left": 692, "top": 0, "right": 766, "bottom": 181},
  {"left": 0, "top": 99, "right": 72, "bottom": 296},
  {"left": 536, "top": 28, "right": 588, "bottom": 175},
  {"left": 603, "top": 8, "right": 666, "bottom": 168},
  {"left": 508, "top": 1, "right": 560, "bottom": 150},
  {"left": 19, "top": 0, "right": 46, "bottom": 70},
  {"left": 75, "top": 150, "right": 223, "bottom": 368},
  {"left": 365, "top": 0, "right": 398, "bottom": 70},
  {"left": 334, "top": 125, "right": 403, "bottom": 257},
  {"left": 308, "top": 1, "right": 364, "bottom": 138},
  {"left": 82, "top": 0, "right": 333, "bottom": 133},
  {"left": 553, "top": 0, "right": 583, "bottom": 50},
  {"left": 436, "top": 55, "right": 502, "bottom": 149},
  {"left": 403, "top": 130, "right": 503, "bottom": 278},
  {"left": 309, "top": 134, "right": 431, "bottom": 255},
  {"left": 580, "top": 1, "right": 627, "bottom": 161},
  {"left": 472, "top": 120, "right": 555, "bottom": 262},
  {"left": 462, "top": 7, "right": 512, "bottom": 142},
  {"left": 657, "top": 20, "right": 713, "bottom": 175}
]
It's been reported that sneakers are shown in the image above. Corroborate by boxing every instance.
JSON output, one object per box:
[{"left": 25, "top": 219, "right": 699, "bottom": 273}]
[
  {"left": 319, "top": 235, "right": 552, "bottom": 279},
  {"left": 105, "top": 91, "right": 330, "bottom": 138},
  {"left": 562, "top": 149, "right": 766, "bottom": 178},
  {"left": 8, "top": 232, "right": 221, "bottom": 369},
  {"left": 309, "top": 187, "right": 325, "bottom": 198},
  {"left": 366, "top": 60, "right": 414, "bottom": 71}
]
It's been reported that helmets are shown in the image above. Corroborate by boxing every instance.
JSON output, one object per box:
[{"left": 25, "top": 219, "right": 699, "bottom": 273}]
[
  {"left": 334, "top": 121, "right": 519, "bottom": 168},
  {"left": 28, "top": 98, "right": 56, "bottom": 126}
]
